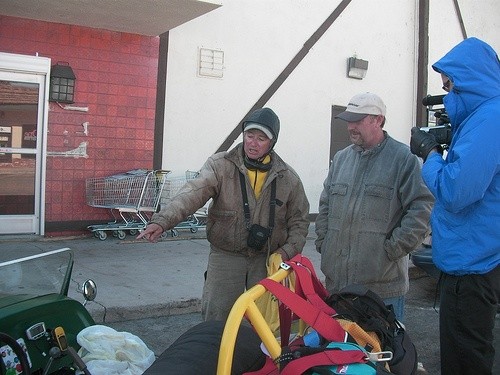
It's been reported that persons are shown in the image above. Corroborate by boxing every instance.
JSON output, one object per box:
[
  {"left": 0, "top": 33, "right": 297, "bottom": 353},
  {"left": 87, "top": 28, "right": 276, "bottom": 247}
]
[
  {"left": 410, "top": 37, "right": 500, "bottom": 375},
  {"left": 315, "top": 93, "right": 434, "bottom": 321},
  {"left": 136, "top": 108, "right": 310, "bottom": 322}
]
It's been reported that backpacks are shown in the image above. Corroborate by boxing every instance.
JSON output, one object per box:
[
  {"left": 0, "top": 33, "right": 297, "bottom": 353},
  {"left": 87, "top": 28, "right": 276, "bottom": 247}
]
[{"left": 327, "top": 288, "right": 419, "bottom": 375}]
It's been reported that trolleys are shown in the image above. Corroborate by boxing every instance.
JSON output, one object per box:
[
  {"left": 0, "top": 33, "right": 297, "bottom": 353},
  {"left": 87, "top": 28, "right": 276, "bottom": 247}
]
[{"left": 84, "top": 167, "right": 214, "bottom": 240}]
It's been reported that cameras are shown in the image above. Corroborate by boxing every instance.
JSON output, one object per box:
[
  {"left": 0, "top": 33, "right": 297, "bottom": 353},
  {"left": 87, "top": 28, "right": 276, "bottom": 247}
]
[{"left": 420, "top": 124, "right": 453, "bottom": 144}]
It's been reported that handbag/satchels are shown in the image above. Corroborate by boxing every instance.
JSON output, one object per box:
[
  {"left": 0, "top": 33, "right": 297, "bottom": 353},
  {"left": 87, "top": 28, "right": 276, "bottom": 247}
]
[{"left": 247, "top": 224, "right": 270, "bottom": 251}]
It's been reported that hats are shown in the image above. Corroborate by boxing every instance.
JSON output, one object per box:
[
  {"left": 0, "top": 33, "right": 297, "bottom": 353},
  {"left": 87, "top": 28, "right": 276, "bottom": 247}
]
[
  {"left": 335, "top": 92, "right": 386, "bottom": 121},
  {"left": 244, "top": 122, "right": 275, "bottom": 140}
]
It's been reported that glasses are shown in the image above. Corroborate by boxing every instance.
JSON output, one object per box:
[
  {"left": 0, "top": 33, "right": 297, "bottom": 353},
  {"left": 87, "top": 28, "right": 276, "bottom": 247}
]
[{"left": 442, "top": 79, "right": 451, "bottom": 92}]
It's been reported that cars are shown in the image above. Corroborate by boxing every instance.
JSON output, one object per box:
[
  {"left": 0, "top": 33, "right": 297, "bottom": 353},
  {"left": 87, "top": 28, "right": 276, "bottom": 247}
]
[{"left": 0, "top": 248, "right": 308, "bottom": 375}]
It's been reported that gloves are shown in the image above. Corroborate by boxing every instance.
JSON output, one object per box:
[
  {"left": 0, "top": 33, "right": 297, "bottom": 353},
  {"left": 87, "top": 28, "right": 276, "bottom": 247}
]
[{"left": 410, "top": 127, "right": 443, "bottom": 163}]
